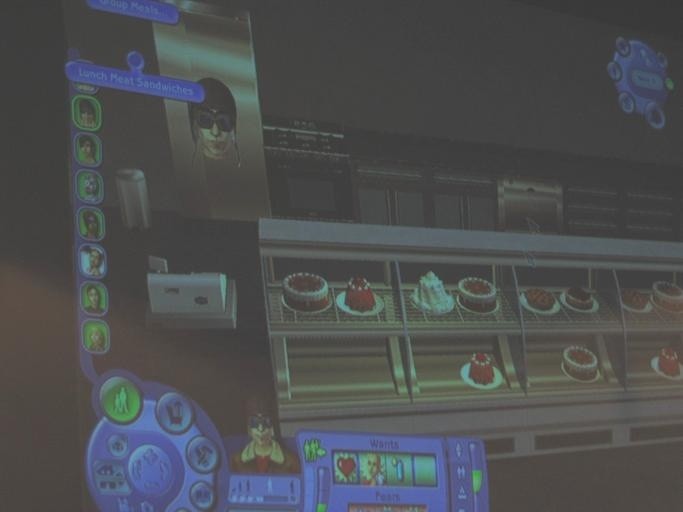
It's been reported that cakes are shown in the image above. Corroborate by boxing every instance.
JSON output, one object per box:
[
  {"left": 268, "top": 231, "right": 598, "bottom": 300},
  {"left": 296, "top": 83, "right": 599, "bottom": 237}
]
[{"left": 282, "top": 271, "right": 683, "bottom": 384}]
